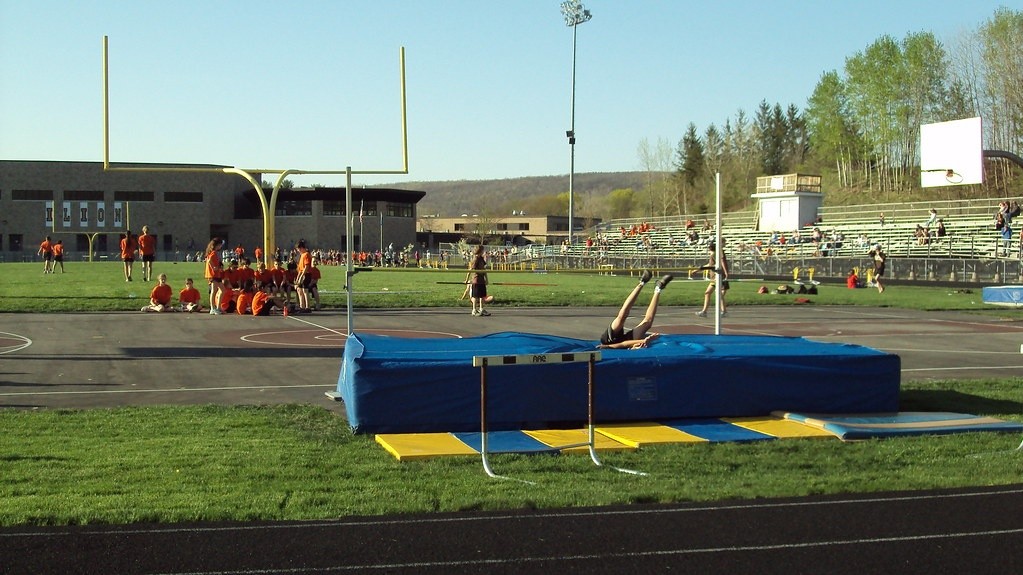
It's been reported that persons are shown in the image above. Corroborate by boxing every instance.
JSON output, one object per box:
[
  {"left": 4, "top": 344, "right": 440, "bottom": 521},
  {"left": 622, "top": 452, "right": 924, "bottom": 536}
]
[
  {"left": 205, "top": 237, "right": 322, "bottom": 316},
  {"left": 352, "top": 242, "right": 449, "bottom": 270},
  {"left": 174, "top": 236, "right": 263, "bottom": 268},
  {"left": 995, "top": 200, "right": 1023, "bottom": 263},
  {"left": 880, "top": 212, "right": 884, "bottom": 228},
  {"left": 37, "top": 235, "right": 66, "bottom": 274},
  {"left": 119, "top": 230, "right": 137, "bottom": 282},
  {"left": 563, "top": 218, "right": 713, "bottom": 255},
  {"left": 595, "top": 269, "right": 674, "bottom": 350},
  {"left": 176, "top": 278, "right": 202, "bottom": 314},
  {"left": 138, "top": 225, "right": 157, "bottom": 282},
  {"left": 914, "top": 208, "right": 945, "bottom": 246},
  {"left": 140, "top": 273, "right": 173, "bottom": 312},
  {"left": 274, "top": 238, "right": 347, "bottom": 266},
  {"left": 458, "top": 245, "right": 494, "bottom": 316},
  {"left": 462, "top": 244, "right": 519, "bottom": 263},
  {"left": 739, "top": 227, "right": 887, "bottom": 294},
  {"left": 691, "top": 237, "right": 730, "bottom": 318}
]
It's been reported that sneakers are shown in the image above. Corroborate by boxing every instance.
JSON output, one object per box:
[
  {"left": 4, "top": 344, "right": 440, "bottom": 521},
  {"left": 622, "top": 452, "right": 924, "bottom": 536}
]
[
  {"left": 639, "top": 269, "right": 653, "bottom": 283},
  {"left": 654, "top": 274, "right": 673, "bottom": 290}
]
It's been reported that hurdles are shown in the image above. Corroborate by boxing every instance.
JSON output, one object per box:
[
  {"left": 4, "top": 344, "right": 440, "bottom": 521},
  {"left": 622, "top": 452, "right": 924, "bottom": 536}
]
[{"left": 472, "top": 350, "right": 651, "bottom": 485}]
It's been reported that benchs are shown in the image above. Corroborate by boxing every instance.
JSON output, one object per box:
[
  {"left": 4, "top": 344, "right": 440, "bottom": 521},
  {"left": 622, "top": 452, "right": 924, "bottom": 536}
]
[{"left": 564, "top": 212, "right": 1023, "bottom": 273}]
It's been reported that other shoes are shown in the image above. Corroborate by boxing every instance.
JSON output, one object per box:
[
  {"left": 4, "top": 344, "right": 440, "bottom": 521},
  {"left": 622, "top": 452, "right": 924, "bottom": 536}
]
[
  {"left": 695, "top": 310, "right": 707, "bottom": 318},
  {"left": 720, "top": 312, "right": 728, "bottom": 318},
  {"left": 479, "top": 309, "right": 491, "bottom": 316},
  {"left": 141, "top": 305, "right": 153, "bottom": 312},
  {"left": 189, "top": 304, "right": 198, "bottom": 313},
  {"left": 210, "top": 308, "right": 226, "bottom": 314},
  {"left": 472, "top": 310, "right": 482, "bottom": 316},
  {"left": 296, "top": 308, "right": 312, "bottom": 313}
]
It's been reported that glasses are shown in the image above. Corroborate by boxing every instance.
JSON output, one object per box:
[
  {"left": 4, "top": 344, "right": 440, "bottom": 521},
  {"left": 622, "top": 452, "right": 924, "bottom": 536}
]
[{"left": 231, "top": 266, "right": 238, "bottom": 269}]
